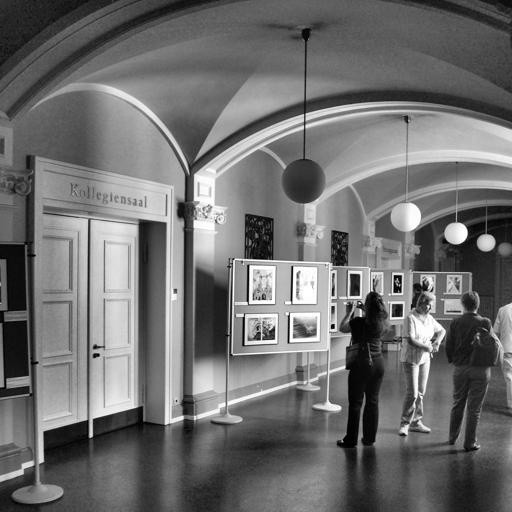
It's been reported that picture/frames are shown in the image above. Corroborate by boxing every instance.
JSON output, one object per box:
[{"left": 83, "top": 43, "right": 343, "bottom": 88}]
[
  {"left": 243, "top": 313, "right": 279, "bottom": 346},
  {"left": 347, "top": 270, "right": 362, "bottom": 300},
  {"left": 331, "top": 270, "right": 337, "bottom": 300},
  {"left": 391, "top": 272, "right": 404, "bottom": 295},
  {"left": 330, "top": 302, "right": 338, "bottom": 333},
  {"left": 370, "top": 271, "right": 384, "bottom": 296},
  {"left": 248, "top": 265, "right": 275, "bottom": 305},
  {"left": 390, "top": 301, "right": 405, "bottom": 320},
  {"left": 287, "top": 312, "right": 321, "bottom": 343},
  {"left": 292, "top": 266, "right": 318, "bottom": 306},
  {"left": 420, "top": 274, "right": 436, "bottom": 295}
]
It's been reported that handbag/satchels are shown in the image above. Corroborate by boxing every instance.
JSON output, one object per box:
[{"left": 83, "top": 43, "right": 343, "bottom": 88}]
[
  {"left": 470, "top": 325, "right": 507, "bottom": 371},
  {"left": 345, "top": 340, "right": 373, "bottom": 371}
]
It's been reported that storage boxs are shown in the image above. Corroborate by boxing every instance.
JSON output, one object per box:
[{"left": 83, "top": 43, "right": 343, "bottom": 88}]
[{"left": 383, "top": 343, "right": 400, "bottom": 352}]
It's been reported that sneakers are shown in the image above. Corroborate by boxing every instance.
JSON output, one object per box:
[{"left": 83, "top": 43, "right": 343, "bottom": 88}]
[
  {"left": 360, "top": 439, "right": 377, "bottom": 448},
  {"left": 410, "top": 419, "right": 432, "bottom": 434},
  {"left": 464, "top": 443, "right": 481, "bottom": 452},
  {"left": 335, "top": 440, "right": 355, "bottom": 451},
  {"left": 398, "top": 423, "right": 409, "bottom": 438}
]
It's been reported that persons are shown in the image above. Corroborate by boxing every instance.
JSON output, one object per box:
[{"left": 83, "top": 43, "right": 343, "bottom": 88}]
[
  {"left": 398, "top": 290, "right": 448, "bottom": 436},
  {"left": 422, "top": 276, "right": 434, "bottom": 292},
  {"left": 493, "top": 301, "right": 512, "bottom": 414},
  {"left": 445, "top": 289, "right": 492, "bottom": 450},
  {"left": 335, "top": 291, "right": 388, "bottom": 449},
  {"left": 411, "top": 283, "right": 423, "bottom": 311}
]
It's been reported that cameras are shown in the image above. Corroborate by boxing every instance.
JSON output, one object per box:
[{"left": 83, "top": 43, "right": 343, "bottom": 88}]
[{"left": 357, "top": 301, "right": 363, "bottom": 308}]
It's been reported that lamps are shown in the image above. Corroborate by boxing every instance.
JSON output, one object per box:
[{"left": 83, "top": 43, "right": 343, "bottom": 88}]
[
  {"left": 390, "top": 115, "right": 421, "bottom": 232},
  {"left": 476, "top": 201, "right": 496, "bottom": 253},
  {"left": 444, "top": 162, "right": 468, "bottom": 245},
  {"left": 497, "top": 211, "right": 512, "bottom": 259},
  {"left": 283, "top": 27, "right": 326, "bottom": 204}
]
